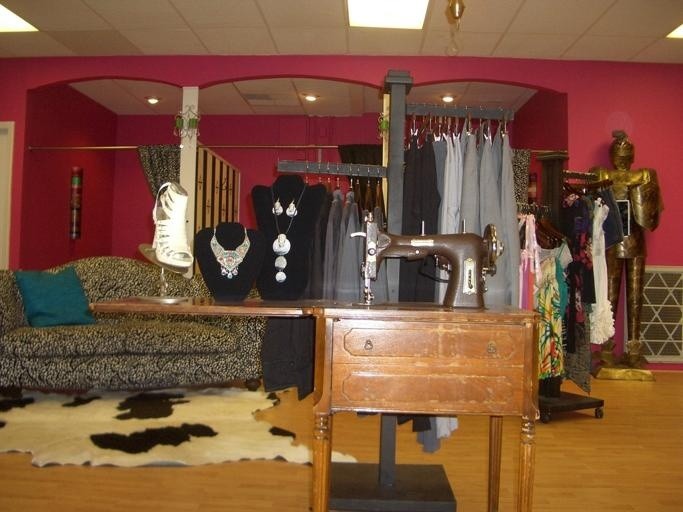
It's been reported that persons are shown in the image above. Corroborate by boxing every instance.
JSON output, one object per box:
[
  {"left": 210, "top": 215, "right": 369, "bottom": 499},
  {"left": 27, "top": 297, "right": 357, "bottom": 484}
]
[{"left": 589, "top": 131, "right": 663, "bottom": 369}]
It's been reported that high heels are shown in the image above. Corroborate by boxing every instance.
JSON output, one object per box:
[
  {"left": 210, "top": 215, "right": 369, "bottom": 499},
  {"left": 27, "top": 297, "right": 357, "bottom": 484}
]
[{"left": 149, "top": 180, "right": 195, "bottom": 269}]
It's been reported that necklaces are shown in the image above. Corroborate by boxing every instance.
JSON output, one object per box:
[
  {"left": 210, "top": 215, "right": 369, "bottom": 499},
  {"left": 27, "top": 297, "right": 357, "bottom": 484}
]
[
  {"left": 210, "top": 227, "right": 251, "bottom": 279},
  {"left": 271, "top": 183, "right": 308, "bottom": 284}
]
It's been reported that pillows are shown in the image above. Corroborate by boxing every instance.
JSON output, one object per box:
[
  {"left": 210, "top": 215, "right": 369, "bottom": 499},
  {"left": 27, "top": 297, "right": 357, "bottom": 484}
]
[{"left": 15, "top": 267, "right": 96, "bottom": 327}]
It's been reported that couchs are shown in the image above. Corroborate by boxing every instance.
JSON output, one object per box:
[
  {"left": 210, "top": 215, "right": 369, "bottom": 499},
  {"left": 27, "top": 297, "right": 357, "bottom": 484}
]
[{"left": 0, "top": 255, "right": 266, "bottom": 391}]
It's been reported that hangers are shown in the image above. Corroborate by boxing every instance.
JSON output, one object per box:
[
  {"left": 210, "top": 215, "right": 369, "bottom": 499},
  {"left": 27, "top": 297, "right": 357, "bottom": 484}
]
[
  {"left": 527, "top": 202, "right": 572, "bottom": 248},
  {"left": 411, "top": 104, "right": 509, "bottom": 132}
]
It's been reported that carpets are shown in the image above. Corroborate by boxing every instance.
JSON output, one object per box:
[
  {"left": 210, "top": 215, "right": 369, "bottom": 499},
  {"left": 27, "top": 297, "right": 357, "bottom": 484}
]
[{"left": 0, "top": 390, "right": 357, "bottom": 468}]
